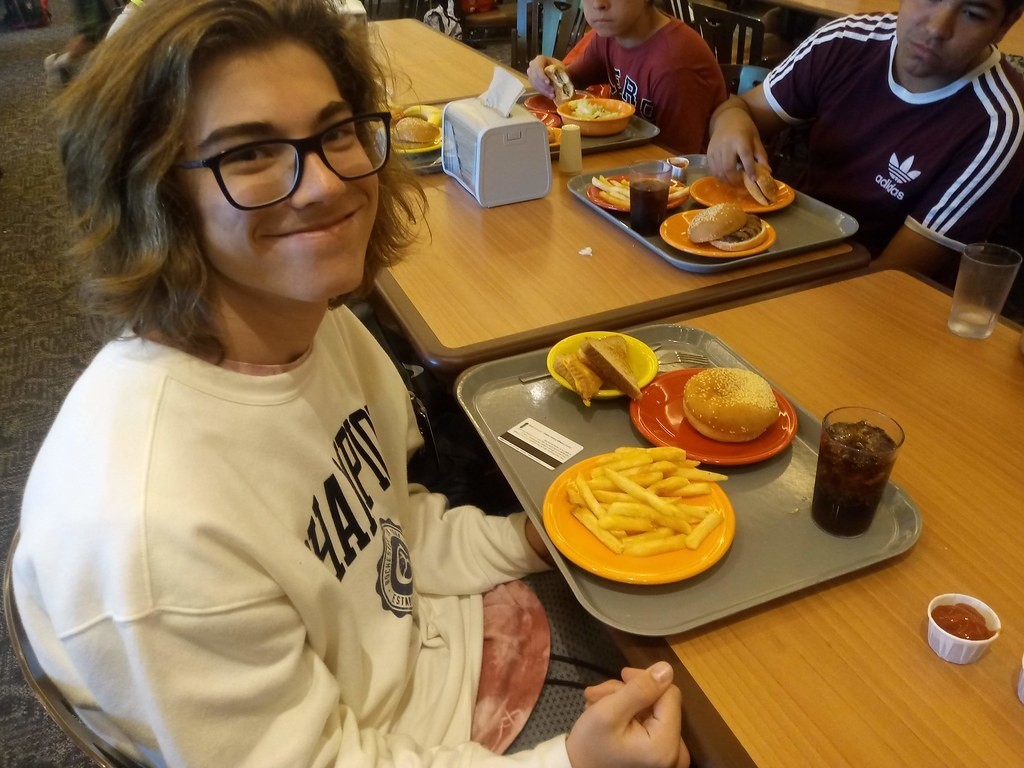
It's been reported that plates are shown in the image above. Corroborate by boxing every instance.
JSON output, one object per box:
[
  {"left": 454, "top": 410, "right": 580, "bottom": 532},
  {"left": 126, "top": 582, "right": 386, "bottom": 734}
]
[
  {"left": 522, "top": 90, "right": 635, "bottom": 151},
  {"left": 630, "top": 368, "right": 798, "bottom": 465},
  {"left": 689, "top": 172, "right": 795, "bottom": 214},
  {"left": 586, "top": 175, "right": 689, "bottom": 215},
  {"left": 389, "top": 124, "right": 442, "bottom": 154},
  {"left": 546, "top": 331, "right": 659, "bottom": 400},
  {"left": 541, "top": 453, "right": 737, "bottom": 587},
  {"left": 659, "top": 209, "right": 776, "bottom": 258}
]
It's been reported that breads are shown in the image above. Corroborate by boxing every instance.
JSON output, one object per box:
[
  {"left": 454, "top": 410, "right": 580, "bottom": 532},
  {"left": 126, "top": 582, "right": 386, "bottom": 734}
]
[
  {"left": 739, "top": 160, "right": 779, "bottom": 207},
  {"left": 394, "top": 117, "right": 440, "bottom": 143},
  {"left": 683, "top": 367, "right": 781, "bottom": 443}
]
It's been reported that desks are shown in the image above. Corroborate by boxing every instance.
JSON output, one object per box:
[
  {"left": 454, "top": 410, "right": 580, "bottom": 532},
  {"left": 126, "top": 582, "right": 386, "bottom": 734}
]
[
  {"left": 370, "top": 19, "right": 534, "bottom": 114},
  {"left": 371, "top": 93, "right": 871, "bottom": 376},
  {"left": 609, "top": 261, "right": 1024, "bottom": 768},
  {"left": 769, "top": 0, "right": 1024, "bottom": 58}
]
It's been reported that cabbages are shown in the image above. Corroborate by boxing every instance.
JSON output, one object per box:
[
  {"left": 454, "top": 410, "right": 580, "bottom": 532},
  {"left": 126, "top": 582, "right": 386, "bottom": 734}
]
[{"left": 568, "top": 97, "right": 626, "bottom": 119}]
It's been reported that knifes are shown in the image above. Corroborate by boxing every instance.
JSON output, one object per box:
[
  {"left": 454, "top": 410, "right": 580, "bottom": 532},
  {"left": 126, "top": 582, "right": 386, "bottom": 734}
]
[{"left": 519, "top": 342, "right": 662, "bottom": 383}]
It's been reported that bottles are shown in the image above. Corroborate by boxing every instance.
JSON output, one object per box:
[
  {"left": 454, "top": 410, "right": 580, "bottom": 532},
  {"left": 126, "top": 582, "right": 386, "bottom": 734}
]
[{"left": 558, "top": 123, "right": 583, "bottom": 176}]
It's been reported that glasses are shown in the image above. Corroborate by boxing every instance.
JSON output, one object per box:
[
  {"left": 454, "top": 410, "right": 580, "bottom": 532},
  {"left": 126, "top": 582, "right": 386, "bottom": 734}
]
[{"left": 172, "top": 111, "right": 392, "bottom": 211}]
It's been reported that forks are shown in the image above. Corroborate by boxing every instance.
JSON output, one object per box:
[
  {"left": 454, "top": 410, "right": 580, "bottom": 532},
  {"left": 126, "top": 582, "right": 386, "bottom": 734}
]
[{"left": 658, "top": 351, "right": 712, "bottom": 366}]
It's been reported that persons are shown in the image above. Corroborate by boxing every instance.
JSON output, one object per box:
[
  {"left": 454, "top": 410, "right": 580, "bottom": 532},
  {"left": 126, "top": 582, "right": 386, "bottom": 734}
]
[
  {"left": 10, "top": 0, "right": 692, "bottom": 768},
  {"left": 526, "top": 0, "right": 727, "bottom": 155},
  {"left": 706, "top": 0, "right": 1024, "bottom": 290}
]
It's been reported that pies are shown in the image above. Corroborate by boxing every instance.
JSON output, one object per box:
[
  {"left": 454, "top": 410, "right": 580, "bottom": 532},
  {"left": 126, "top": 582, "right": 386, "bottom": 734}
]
[{"left": 543, "top": 65, "right": 576, "bottom": 107}]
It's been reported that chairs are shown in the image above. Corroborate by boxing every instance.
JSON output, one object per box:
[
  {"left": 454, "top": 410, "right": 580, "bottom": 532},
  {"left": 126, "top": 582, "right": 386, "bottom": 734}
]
[
  {"left": 453, "top": 0, "right": 589, "bottom": 74},
  {"left": 655, "top": 0, "right": 793, "bottom": 170},
  {"left": 2, "top": 524, "right": 145, "bottom": 768}
]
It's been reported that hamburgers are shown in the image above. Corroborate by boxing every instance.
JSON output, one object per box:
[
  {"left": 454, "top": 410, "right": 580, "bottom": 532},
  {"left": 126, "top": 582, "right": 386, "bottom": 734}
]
[{"left": 688, "top": 201, "right": 768, "bottom": 251}]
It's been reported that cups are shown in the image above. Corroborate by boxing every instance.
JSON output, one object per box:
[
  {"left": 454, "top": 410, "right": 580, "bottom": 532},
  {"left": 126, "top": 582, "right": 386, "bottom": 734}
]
[
  {"left": 667, "top": 157, "right": 689, "bottom": 183},
  {"left": 626, "top": 158, "right": 673, "bottom": 238},
  {"left": 811, "top": 405, "right": 905, "bottom": 540},
  {"left": 948, "top": 242, "right": 1023, "bottom": 340}
]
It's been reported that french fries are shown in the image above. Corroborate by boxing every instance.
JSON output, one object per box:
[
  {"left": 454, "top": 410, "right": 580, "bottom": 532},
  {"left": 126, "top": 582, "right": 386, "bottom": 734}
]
[
  {"left": 565, "top": 445, "right": 729, "bottom": 555},
  {"left": 592, "top": 173, "right": 691, "bottom": 206}
]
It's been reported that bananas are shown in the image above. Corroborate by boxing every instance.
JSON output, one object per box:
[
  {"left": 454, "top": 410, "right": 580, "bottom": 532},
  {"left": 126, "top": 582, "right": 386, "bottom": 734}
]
[{"left": 401, "top": 104, "right": 443, "bottom": 127}]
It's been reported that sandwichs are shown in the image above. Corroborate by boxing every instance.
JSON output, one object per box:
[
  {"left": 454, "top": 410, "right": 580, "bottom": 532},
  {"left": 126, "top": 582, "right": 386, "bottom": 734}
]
[
  {"left": 577, "top": 334, "right": 644, "bottom": 401},
  {"left": 553, "top": 355, "right": 603, "bottom": 406}
]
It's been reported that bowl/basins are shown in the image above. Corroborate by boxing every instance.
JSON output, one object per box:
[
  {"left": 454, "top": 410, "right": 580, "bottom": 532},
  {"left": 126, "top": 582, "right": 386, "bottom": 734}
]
[
  {"left": 1017, "top": 651, "right": 1024, "bottom": 703},
  {"left": 557, "top": 98, "right": 635, "bottom": 136},
  {"left": 928, "top": 593, "right": 1002, "bottom": 665}
]
